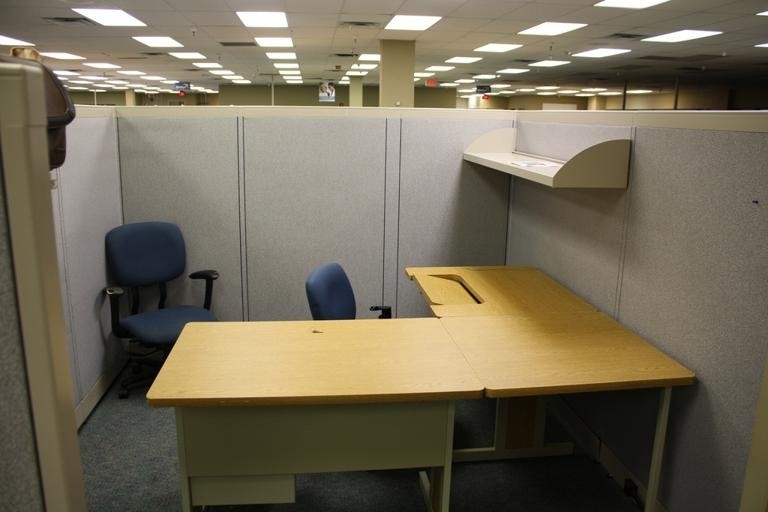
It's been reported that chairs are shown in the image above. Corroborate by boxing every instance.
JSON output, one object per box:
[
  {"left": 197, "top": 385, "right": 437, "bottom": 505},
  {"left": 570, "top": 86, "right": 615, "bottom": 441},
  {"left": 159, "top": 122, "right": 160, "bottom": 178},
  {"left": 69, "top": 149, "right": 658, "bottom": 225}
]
[
  {"left": 102, "top": 220, "right": 220, "bottom": 401},
  {"left": 306, "top": 263, "right": 393, "bottom": 320}
]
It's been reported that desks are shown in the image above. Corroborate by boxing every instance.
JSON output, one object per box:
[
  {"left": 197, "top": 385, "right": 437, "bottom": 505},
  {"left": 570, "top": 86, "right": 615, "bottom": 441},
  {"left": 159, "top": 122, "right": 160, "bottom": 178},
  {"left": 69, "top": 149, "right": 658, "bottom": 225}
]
[
  {"left": 403, "top": 266, "right": 698, "bottom": 511},
  {"left": 147, "top": 317, "right": 483, "bottom": 512}
]
